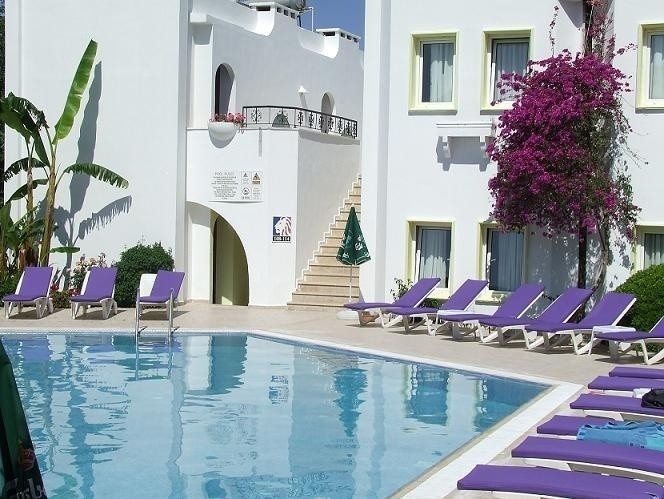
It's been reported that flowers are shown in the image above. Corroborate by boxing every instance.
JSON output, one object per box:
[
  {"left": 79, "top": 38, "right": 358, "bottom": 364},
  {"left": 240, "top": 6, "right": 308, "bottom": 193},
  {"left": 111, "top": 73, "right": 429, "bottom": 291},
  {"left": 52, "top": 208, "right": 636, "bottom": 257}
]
[{"left": 212, "top": 113, "right": 245, "bottom": 122}]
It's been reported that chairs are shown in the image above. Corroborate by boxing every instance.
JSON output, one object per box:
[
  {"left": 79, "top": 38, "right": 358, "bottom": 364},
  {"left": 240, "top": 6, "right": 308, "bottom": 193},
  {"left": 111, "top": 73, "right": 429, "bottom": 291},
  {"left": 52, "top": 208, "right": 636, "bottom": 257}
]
[
  {"left": 588, "top": 375, "right": 663, "bottom": 398},
  {"left": 526, "top": 290, "right": 635, "bottom": 356},
  {"left": 595, "top": 316, "right": 663, "bottom": 366},
  {"left": 387, "top": 279, "right": 489, "bottom": 336},
  {"left": 457, "top": 464, "right": 663, "bottom": 498},
  {"left": 444, "top": 283, "right": 546, "bottom": 343},
  {"left": 609, "top": 365, "right": 664, "bottom": 380},
  {"left": 344, "top": 277, "right": 440, "bottom": 327},
  {"left": 481, "top": 287, "right": 593, "bottom": 350},
  {"left": 2, "top": 266, "right": 55, "bottom": 319},
  {"left": 511, "top": 435, "right": 664, "bottom": 487},
  {"left": 570, "top": 392, "right": 663, "bottom": 427},
  {"left": 537, "top": 413, "right": 663, "bottom": 451},
  {"left": 134, "top": 270, "right": 185, "bottom": 320},
  {"left": 69, "top": 266, "right": 118, "bottom": 320}
]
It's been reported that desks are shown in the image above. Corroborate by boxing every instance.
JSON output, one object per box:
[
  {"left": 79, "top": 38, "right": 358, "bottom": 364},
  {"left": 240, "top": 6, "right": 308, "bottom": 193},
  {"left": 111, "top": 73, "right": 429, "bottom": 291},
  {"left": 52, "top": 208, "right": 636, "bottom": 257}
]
[
  {"left": 589, "top": 326, "right": 638, "bottom": 357},
  {"left": 432, "top": 309, "right": 485, "bottom": 341}
]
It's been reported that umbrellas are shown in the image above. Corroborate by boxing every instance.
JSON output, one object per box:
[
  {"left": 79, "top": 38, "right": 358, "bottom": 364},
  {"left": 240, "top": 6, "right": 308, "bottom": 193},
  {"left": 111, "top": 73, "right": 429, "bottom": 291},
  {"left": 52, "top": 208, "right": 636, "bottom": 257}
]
[
  {"left": 328, "top": 355, "right": 370, "bottom": 443},
  {"left": 334, "top": 204, "right": 371, "bottom": 301}
]
